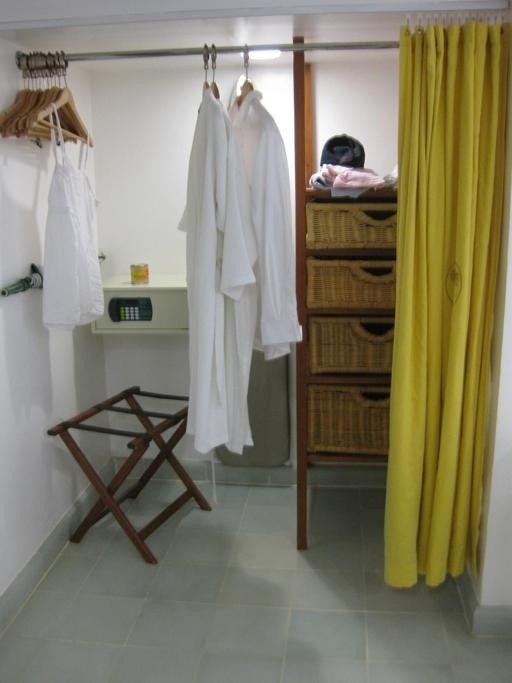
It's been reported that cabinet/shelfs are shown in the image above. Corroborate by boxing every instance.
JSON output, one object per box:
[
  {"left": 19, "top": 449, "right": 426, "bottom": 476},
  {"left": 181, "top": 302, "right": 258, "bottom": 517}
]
[{"left": 305, "top": 190, "right": 399, "bottom": 463}]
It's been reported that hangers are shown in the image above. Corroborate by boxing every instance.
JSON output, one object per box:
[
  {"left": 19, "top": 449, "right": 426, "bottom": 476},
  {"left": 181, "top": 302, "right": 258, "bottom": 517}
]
[
  {"left": 202, "top": 44, "right": 253, "bottom": 108},
  {"left": 0, "top": 51, "right": 94, "bottom": 145}
]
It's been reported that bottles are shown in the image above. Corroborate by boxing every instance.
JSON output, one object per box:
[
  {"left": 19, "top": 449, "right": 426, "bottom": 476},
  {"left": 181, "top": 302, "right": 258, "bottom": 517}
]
[{"left": 131, "top": 264, "right": 149, "bottom": 285}]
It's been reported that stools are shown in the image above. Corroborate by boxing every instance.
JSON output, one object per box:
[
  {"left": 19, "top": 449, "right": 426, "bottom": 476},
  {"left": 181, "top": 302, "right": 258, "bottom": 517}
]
[{"left": 49, "top": 386, "right": 212, "bottom": 564}]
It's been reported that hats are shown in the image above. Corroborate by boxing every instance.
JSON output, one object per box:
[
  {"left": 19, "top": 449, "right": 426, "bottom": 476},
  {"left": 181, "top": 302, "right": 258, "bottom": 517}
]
[{"left": 320, "top": 134, "right": 365, "bottom": 168}]
